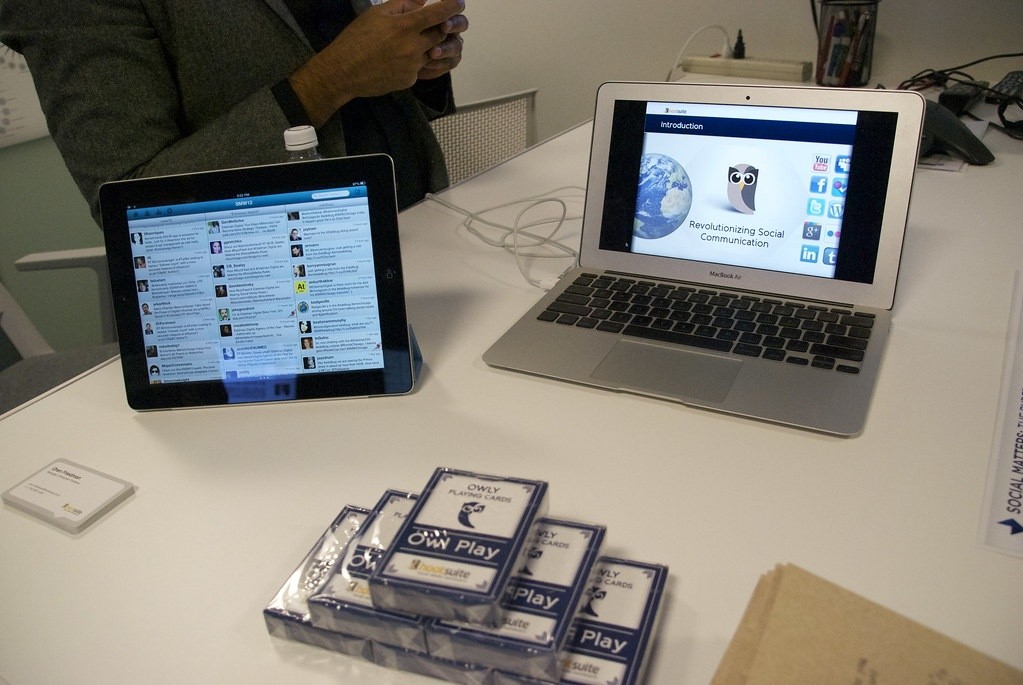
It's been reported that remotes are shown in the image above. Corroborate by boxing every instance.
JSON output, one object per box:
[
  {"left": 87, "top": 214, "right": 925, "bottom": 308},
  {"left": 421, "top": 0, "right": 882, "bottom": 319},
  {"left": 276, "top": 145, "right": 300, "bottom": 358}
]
[
  {"left": 938, "top": 81, "right": 989, "bottom": 119},
  {"left": 984, "top": 70, "right": 1023, "bottom": 104}
]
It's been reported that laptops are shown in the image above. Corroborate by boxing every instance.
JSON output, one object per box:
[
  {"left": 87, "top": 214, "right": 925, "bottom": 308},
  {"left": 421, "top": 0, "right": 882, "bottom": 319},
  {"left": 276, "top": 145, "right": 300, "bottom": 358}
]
[{"left": 481, "top": 83, "right": 926, "bottom": 439}]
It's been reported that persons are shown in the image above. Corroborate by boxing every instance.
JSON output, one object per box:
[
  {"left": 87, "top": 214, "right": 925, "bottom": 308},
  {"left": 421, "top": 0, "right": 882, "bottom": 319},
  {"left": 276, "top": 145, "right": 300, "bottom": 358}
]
[
  {"left": 131, "top": 220, "right": 316, "bottom": 375},
  {"left": 1, "top": 0, "right": 468, "bottom": 213}
]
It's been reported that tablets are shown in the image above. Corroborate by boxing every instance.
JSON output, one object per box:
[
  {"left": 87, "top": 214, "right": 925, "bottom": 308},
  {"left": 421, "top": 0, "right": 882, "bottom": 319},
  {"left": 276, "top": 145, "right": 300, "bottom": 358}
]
[{"left": 95, "top": 154, "right": 416, "bottom": 412}]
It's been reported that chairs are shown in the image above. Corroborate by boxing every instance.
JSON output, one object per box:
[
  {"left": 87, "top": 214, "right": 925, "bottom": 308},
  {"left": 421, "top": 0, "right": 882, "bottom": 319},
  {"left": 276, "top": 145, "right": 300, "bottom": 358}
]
[
  {"left": 431, "top": 90, "right": 539, "bottom": 188},
  {"left": 0, "top": 247, "right": 121, "bottom": 416}
]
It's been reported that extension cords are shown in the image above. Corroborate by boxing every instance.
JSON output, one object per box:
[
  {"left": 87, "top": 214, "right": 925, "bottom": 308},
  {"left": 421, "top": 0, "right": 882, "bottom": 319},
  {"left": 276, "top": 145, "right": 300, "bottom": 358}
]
[{"left": 682, "top": 54, "right": 813, "bottom": 82}]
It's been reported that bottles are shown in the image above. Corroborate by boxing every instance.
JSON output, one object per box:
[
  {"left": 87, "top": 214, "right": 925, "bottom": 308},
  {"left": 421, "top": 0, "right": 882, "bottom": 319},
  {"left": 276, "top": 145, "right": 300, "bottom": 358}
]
[{"left": 283, "top": 126, "right": 323, "bottom": 164}]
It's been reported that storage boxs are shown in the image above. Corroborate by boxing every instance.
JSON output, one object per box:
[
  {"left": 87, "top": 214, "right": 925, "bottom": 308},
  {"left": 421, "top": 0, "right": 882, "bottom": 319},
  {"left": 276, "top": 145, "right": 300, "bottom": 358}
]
[{"left": 264, "top": 466, "right": 667, "bottom": 685}]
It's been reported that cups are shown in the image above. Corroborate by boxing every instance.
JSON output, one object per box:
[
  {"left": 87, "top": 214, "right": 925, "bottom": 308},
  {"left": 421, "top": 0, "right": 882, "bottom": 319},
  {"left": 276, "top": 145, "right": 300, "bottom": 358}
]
[{"left": 815, "top": 0, "right": 881, "bottom": 87}]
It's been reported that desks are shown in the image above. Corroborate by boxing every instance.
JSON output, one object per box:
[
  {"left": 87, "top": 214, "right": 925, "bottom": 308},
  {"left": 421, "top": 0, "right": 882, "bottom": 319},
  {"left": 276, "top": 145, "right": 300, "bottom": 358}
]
[{"left": 0, "top": 94, "right": 1023, "bottom": 685}]
